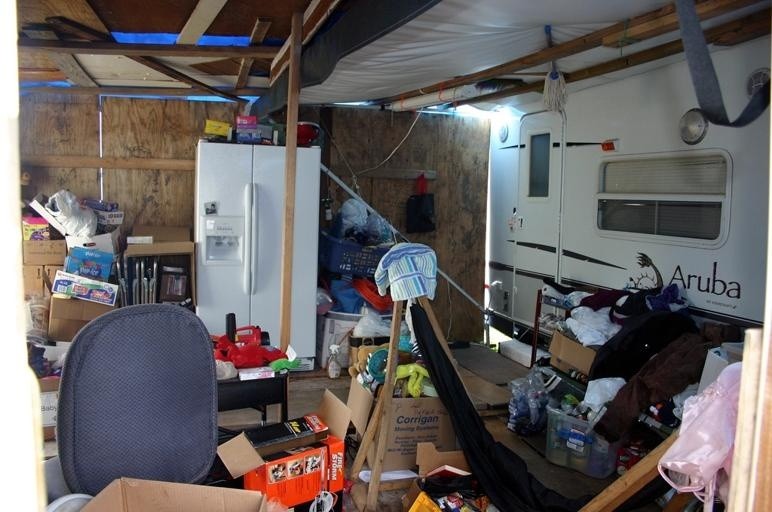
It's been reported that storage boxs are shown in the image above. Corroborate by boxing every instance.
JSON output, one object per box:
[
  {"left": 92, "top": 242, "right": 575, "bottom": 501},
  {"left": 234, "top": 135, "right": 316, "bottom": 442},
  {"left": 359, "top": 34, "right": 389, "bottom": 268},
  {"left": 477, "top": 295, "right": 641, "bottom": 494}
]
[
  {"left": 695, "top": 342, "right": 744, "bottom": 395},
  {"left": 399, "top": 441, "right": 502, "bottom": 511},
  {"left": 545, "top": 400, "right": 615, "bottom": 482},
  {"left": 549, "top": 328, "right": 596, "bottom": 381},
  {"left": 22, "top": 193, "right": 204, "bottom": 341},
  {"left": 81, "top": 476, "right": 268, "bottom": 511},
  {"left": 36, "top": 378, "right": 61, "bottom": 444},
  {"left": 215, "top": 386, "right": 354, "bottom": 509},
  {"left": 344, "top": 365, "right": 458, "bottom": 476}
]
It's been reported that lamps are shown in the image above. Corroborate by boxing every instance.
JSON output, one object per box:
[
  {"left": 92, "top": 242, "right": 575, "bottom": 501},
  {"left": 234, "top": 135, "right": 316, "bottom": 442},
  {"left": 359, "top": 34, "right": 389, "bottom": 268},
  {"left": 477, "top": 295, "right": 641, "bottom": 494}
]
[{"left": 392, "top": 86, "right": 476, "bottom": 112}]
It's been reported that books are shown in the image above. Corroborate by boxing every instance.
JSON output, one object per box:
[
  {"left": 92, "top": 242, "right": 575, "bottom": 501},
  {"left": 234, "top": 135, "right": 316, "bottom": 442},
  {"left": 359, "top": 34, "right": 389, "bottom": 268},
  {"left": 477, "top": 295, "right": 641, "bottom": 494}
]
[{"left": 425, "top": 465, "right": 470, "bottom": 480}]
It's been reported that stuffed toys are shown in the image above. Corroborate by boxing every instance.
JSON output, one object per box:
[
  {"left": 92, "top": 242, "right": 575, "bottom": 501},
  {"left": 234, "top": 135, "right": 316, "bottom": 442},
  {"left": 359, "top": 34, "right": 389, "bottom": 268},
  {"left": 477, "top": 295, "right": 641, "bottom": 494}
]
[{"left": 349, "top": 344, "right": 429, "bottom": 397}]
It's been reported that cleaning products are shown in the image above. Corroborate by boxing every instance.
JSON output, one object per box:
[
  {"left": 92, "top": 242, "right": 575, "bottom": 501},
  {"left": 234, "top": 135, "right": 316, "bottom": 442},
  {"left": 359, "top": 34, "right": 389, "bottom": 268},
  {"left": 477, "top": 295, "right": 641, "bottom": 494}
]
[{"left": 327, "top": 344, "right": 343, "bottom": 379}]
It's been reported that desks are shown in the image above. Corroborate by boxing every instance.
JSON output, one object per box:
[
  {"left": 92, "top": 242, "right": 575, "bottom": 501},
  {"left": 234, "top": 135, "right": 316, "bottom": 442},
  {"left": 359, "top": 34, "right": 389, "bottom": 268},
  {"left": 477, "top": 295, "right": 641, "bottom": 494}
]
[{"left": 217, "top": 366, "right": 289, "bottom": 426}]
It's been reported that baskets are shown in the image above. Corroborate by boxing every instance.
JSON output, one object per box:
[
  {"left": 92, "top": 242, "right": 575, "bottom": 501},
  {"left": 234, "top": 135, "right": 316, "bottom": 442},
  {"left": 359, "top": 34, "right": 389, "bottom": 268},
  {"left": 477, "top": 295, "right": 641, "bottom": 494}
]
[{"left": 319, "top": 228, "right": 390, "bottom": 277}]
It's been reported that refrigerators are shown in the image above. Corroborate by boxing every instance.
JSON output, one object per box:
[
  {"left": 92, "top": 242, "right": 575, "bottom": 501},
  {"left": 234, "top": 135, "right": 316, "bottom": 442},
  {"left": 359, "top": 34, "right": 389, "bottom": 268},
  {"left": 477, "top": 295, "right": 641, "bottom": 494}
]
[{"left": 194, "top": 139, "right": 322, "bottom": 372}]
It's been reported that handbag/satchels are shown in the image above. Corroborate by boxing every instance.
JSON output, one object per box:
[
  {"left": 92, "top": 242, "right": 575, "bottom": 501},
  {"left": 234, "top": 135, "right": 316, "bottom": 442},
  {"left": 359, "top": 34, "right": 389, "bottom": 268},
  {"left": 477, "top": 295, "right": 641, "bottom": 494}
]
[
  {"left": 407, "top": 175, "right": 435, "bottom": 233},
  {"left": 44, "top": 189, "right": 97, "bottom": 243}
]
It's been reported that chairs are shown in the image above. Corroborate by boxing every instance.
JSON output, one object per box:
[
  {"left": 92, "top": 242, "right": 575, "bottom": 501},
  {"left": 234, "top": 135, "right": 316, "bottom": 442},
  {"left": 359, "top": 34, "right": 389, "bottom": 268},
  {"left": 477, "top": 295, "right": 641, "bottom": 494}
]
[{"left": 39, "top": 301, "right": 218, "bottom": 505}]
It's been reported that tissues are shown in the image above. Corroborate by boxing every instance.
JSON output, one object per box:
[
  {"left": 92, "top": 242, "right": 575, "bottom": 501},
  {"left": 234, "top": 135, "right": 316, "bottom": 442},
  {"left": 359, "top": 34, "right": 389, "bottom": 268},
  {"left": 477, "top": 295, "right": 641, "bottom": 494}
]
[
  {"left": 237, "top": 99, "right": 259, "bottom": 129},
  {"left": 204, "top": 118, "right": 235, "bottom": 142}
]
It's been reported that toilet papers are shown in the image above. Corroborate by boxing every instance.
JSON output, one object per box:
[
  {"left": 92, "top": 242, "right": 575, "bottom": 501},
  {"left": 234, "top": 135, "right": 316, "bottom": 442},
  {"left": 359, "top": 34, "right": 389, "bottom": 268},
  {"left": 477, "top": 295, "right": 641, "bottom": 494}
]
[{"left": 543, "top": 283, "right": 566, "bottom": 302}]
